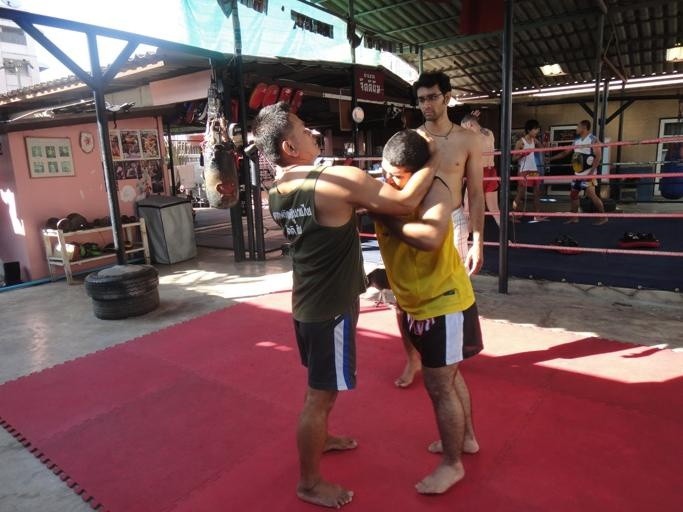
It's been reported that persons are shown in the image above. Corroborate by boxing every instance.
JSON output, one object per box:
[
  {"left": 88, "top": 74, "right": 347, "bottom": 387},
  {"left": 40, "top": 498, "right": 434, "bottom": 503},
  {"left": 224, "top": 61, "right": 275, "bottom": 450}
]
[
  {"left": 461, "top": 109, "right": 513, "bottom": 245},
  {"left": 543, "top": 120, "right": 608, "bottom": 227},
  {"left": 363, "top": 129, "right": 484, "bottom": 494},
  {"left": 512, "top": 119, "right": 550, "bottom": 223},
  {"left": 392, "top": 69, "right": 485, "bottom": 388},
  {"left": 250, "top": 101, "right": 443, "bottom": 509}
]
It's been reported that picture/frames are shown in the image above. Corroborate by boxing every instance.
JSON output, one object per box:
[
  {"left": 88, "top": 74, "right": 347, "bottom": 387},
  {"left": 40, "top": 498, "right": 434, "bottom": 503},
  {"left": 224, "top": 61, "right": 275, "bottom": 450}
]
[
  {"left": 23, "top": 135, "right": 76, "bottom": 179},
  {"left": 78, "top": 130, "right": 95, "bottom": 155}
]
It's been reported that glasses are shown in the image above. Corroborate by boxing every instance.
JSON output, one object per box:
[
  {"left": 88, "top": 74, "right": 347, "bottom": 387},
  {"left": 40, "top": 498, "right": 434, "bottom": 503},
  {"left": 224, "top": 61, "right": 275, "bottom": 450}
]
[{"left": 416, "top": 93, "right": 443, "bottom": 103}]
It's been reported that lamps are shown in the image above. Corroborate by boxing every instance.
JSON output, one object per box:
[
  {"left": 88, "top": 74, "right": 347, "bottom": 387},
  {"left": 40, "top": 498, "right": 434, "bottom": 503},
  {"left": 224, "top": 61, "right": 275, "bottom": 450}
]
[{"left": 665, "top": 41, "right": 683, "bottom": 63}]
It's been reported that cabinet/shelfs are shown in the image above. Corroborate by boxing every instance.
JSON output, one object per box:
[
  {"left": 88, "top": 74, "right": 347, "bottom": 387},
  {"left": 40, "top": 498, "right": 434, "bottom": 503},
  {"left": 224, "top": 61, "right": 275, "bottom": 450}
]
[{"left": 39, "top": 215, "right": 150, "bottom": 286}]
